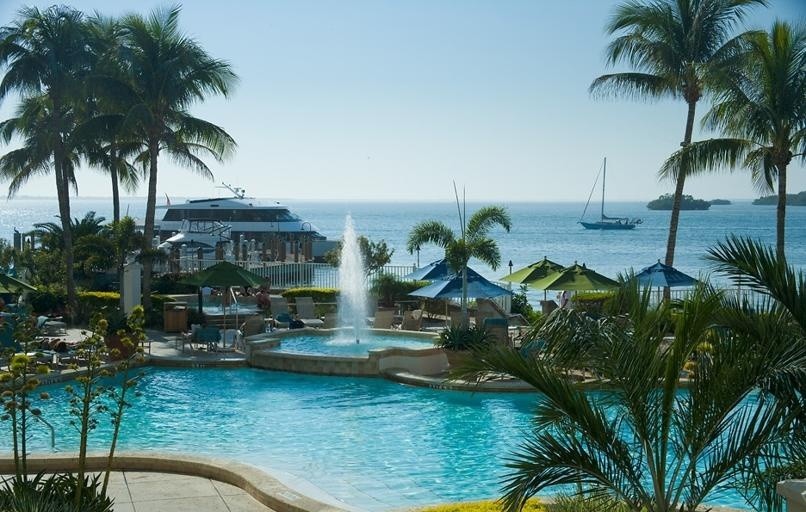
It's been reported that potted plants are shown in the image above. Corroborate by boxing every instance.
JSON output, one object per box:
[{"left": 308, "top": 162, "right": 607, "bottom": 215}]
[
  {"left": 434, "top": 325, "right": 498, "bottom": 370},
  {"left": 104, "top": 306, "right": 144, "bottom": 361}
]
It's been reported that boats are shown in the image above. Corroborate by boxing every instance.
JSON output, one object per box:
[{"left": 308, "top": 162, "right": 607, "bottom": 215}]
[
  {"left": 157, "top": 187, "right": 326, "bottom": 250},
  {"left": 155, "top": 218, "right": 232, "bottom": 260}
]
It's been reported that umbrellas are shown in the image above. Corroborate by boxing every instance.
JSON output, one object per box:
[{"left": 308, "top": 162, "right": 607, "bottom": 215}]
[
  {"left": 498, "top": 254, "right": 566, "bottom": 301},
  {"left": 406, "top": 278, "right": 517, "bottom": 309},
  {"left": 0, "top": 273, "right": 38, "bottom": 295},
  {"left": 179, "top": 259, "right": 268, "bottom": 349},
  {"left": 528, "top": 260, "right": 621, "bottom": 294},
  {"left": 624, "top": 258, "right": 710, "bottom": 337},
  {"left": 402, "top": 259, "right": 480, "bottom": 326}
]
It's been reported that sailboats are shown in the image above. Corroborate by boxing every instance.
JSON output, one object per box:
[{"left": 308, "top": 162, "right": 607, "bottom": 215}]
[{"left": 580, "top": 157, "right": 639, "bottom": 230}]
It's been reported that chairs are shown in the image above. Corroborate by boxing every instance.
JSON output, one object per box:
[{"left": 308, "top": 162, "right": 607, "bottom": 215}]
[
  {"left": 540, "top": 300, "right": 558, "bottom": 314},
  {"left": 294, "top": 298, "right": 324, "bottom": 328},
  {"left": 476, "top": 298, "right": 531, "bottom": 344},
  {"left": 400, "top": 304, "right": 426, "bottom": 331},
  {"left": 235, "top": 297, "right": 290, "bottom": 352},
  {"left": 175, "top": 324, "right": 221, "bottom": 354},
  {"left": 36, "top": 314, "right": 67, "bottom": 336},
  {"left": 365, "top": 306, "right": 396, "bottom": 328}
]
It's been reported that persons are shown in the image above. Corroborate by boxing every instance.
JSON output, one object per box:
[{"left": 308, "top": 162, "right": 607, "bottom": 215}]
[
  {"left": 234, "top": 315, "right": 249, "bottom": 336},
  {"left": 559, "top": 289, "right": 571, "bottom": 309},
  {"left": 289, "top": 312, "right": 304, "bottom": 329},
  {"left": 41, "top": 336, "right": 67, "bottom": 353},
  {"left": 201, "top": 277, "right": 271, "bottom": 310},
  {"left": 180, "top": 317, "right": 208, "bottom": 338}
]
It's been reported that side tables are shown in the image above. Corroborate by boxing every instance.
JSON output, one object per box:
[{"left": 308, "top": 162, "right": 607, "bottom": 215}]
[{"left": 138, "top": 339, "right": 152, "bottom": 358}]
